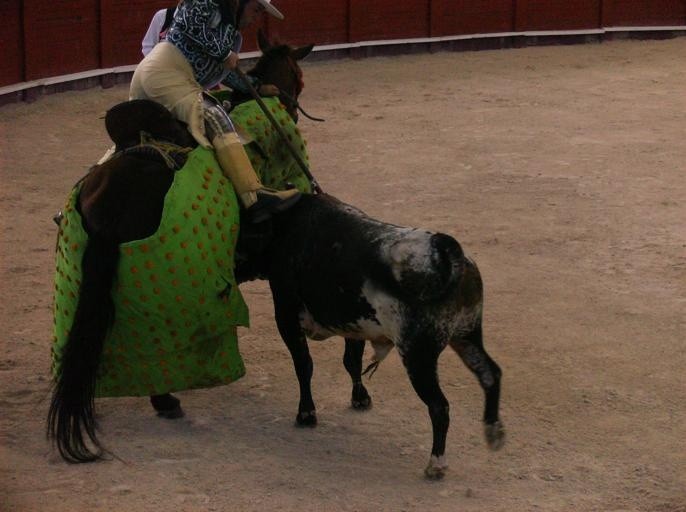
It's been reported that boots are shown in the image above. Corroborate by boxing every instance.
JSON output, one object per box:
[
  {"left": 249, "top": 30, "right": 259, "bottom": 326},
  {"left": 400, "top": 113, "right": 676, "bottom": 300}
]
[{"left": 214, "top": 132, "right": 300, "bottom": 223}]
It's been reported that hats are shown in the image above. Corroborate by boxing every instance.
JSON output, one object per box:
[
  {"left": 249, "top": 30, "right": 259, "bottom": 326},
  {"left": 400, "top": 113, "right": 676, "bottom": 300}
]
[{"left": 258, "top": 0, "right": 284, "bottom": 20}]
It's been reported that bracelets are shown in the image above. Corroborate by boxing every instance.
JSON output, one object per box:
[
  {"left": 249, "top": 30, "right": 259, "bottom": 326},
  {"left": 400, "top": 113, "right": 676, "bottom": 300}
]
[{"left": 222, "top": 50, "right": 233, "bottom": 61}]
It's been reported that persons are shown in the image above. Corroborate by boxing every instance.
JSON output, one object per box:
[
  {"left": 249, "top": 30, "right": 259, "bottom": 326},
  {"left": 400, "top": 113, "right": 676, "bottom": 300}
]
[
  {"left": 141, "top": 6, "right": 178, "bottom": 57},
  {"left": 98, "top": 0, "right": 302, "bottom": 223}
]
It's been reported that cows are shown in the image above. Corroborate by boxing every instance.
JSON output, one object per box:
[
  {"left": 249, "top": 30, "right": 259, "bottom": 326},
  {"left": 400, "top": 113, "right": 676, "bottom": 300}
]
[{"left": 235, "top": 186, "right": 507, "bottom": 481}]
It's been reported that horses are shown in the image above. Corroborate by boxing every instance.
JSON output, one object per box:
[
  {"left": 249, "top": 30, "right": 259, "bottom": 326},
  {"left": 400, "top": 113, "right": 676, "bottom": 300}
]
[{"left": 42, "top": 24, "right": 316, "bottom": 464}]
